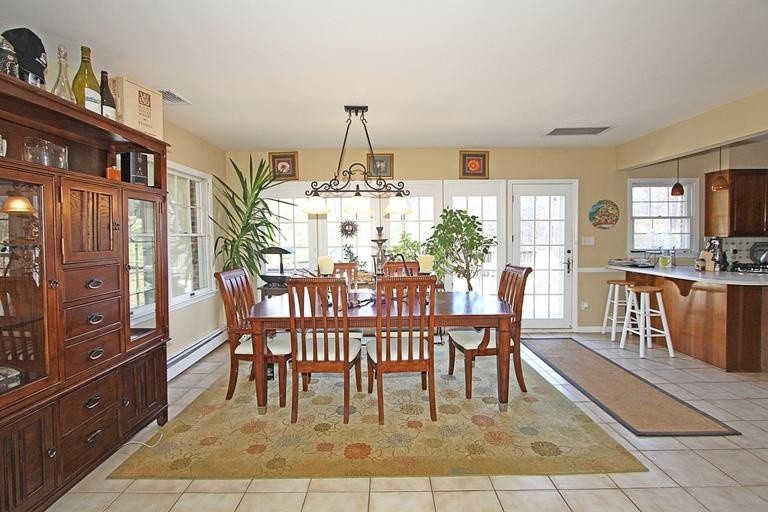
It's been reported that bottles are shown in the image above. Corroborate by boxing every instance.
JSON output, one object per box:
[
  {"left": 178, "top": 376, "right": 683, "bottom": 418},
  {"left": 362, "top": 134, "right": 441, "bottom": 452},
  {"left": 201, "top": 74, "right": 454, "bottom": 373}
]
[
  {"left": 96, "top": 69, "right": 116, "bottom": 121},
  {"left": 51, "top": 45, "right": 78, "bottom": 104},
  {"left": 72, "top": 46, "right": 101, "bottom": 114},
  {"left": 695, "top": 258, "right": 706, "bottom": 271}
]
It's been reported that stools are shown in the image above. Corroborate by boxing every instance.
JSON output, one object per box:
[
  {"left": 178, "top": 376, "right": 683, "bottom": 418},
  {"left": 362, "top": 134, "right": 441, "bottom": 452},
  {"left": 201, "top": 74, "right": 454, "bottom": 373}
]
[
  {"left": 617, "top": 284, "right": 678, "bottom": 361},
  {"left": 601, "top": 278, "right": 640, "bottom": 343}
]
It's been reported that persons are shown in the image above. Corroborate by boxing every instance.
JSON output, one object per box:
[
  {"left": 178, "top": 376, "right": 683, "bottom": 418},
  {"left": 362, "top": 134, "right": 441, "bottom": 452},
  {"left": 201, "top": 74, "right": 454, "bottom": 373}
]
[{"left": 376, "top": 160, "right": 385, "bottom": 174}]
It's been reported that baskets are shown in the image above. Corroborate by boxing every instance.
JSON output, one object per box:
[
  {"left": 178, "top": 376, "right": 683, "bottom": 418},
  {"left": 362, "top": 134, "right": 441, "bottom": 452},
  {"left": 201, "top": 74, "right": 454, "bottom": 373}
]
[{"left": 352, "top": 271, "right": 372, "bottom": 282}]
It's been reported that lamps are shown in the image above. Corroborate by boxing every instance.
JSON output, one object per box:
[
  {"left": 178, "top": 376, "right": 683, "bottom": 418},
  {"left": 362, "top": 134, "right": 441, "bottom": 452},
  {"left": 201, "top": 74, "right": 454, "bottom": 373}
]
[
  {"left": 668, "top": 156, "right": 685, "bottom": 198},
  {"left": 709, "top": 145, "right": 732, "bottom": 193},
  {"left": 2, "top": 180, "right": 36, "bottom": 218},
  {"left": 303, "top": 105, "right": 413, "bottom": 219},
  {"left": 258, "top": 247, "right": 294, "bottom": 281}
]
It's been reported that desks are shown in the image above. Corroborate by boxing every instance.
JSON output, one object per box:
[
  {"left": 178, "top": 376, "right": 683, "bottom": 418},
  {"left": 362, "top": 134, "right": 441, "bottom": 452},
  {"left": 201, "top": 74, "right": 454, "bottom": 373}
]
[
  {"left": 605, "top": 262, "right": 767, "bottom": 373},
  {"left": 256, "top": 284, "right": 292, "bottom": 334},
  {"left": 241, "top": 290, "right": 519, "bottom": 416}
]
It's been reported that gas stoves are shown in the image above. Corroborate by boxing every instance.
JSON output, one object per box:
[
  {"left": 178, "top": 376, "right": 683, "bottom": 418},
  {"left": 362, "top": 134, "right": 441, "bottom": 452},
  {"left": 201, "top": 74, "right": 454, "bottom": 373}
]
[{"left": 726, "top": 262, "right": 768, "bottom": 273}]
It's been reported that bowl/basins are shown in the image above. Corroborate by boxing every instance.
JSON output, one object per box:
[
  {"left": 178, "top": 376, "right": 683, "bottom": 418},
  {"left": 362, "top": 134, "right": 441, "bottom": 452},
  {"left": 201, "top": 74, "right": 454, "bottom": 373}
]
[{"left": 260, "top": 274, "right": 290, "bottom": 284}]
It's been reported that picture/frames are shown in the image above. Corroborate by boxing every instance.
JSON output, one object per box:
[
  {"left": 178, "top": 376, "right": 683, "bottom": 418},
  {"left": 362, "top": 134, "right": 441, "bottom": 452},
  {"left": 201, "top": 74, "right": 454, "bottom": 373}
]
[
  {"left": 365, "top": 152, "right": 396, "bottom": 181},
  {"left": 458, "top": 150, "right": 491, "bottom": 182},
  {"left": 267, "top": 149, "right": 302, "bottom": 183}
]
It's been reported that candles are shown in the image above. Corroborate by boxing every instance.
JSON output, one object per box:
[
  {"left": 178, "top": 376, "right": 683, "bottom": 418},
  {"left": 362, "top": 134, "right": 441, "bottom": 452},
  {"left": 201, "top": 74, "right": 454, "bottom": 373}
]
[
  {"left": 317, "top": 255, "right": 335, "bottom": 276},
  {"left": 415, "top": 253, "right": 435, "bottom": 274}
]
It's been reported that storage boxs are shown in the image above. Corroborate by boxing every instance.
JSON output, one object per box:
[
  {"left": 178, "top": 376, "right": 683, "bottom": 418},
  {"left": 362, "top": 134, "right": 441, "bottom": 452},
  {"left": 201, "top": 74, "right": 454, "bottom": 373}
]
[{"left": 101, "top": 74, "right": 166, "bottom": 145}]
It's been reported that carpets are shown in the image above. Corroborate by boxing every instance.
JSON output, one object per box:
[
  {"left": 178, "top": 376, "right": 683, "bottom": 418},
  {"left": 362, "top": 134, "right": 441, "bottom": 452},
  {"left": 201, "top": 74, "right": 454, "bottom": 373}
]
[
  {"left": 516, "top": 335, "right": 746, "bottom": 438},
  {"left": 104, "top": 338, "right": 654, "bottom": 482}
]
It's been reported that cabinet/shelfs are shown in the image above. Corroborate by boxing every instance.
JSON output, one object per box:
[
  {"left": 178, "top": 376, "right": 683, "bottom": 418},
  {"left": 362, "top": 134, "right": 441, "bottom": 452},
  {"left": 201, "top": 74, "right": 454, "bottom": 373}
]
[
  {"left": 702, "top": 167, "right": 767, "bottom": 239},
  {"left": 0, "top": 71, "right": 173, "bottom": 511}
]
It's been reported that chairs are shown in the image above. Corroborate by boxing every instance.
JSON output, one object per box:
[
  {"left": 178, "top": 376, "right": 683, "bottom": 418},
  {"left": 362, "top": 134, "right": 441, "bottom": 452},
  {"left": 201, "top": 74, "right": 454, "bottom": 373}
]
[
  {"left": 284, "top": 275, "right": 366, "bottom": 425},
  {"left": 314, "top": 260, "right": 361, "bottom": 289},
  {"left": 445, "top": 261, "right": 534, "bottom": 402},
  {"left": 365, "top": 274, "right": 442, "bottom": 428},
  {"left": 381, "top": 259, "right": 424, "bottom": 286},
  {"left": 210, "top": 265, "right": 308, "bottom": 409}
]
